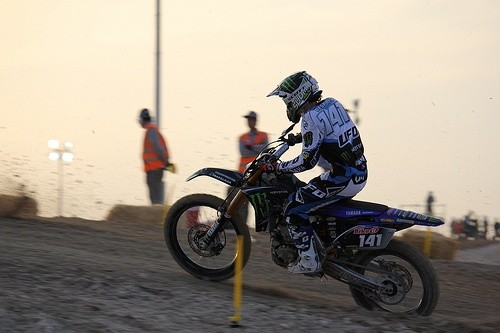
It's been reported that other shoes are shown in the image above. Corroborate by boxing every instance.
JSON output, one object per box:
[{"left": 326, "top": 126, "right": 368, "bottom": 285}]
[{"left": 285, "top": 260, "right": 323, "bottom": 275}]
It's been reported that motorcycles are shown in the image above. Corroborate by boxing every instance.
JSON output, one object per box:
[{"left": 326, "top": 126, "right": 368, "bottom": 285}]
[{"left": 164, "top": 121, "right": 440, "bottom": 316}]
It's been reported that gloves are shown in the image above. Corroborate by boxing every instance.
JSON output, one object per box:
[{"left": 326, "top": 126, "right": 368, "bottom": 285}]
[{"left": 257, "top": 160, "right": 284, "bottom": 175}]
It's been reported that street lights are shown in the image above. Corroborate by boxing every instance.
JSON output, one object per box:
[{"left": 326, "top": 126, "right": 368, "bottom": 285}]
[{"left": 48, "top": 139, "right": 74, "bottom": 218}]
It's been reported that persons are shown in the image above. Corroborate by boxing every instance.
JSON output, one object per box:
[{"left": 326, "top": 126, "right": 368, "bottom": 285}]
[
  {"left": 239, "top": 111, "right": 268, "bottom": 175},
  {"left": 261, "top": 71, "right": 368, "bottom": 275},
  {"left": 139, "top": 109, "right": 175, "bottom": 204}
]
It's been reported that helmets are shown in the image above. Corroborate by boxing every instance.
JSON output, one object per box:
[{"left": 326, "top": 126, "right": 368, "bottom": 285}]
[{"left": 267, "top": 71, "right": 323, "bottom": 125}]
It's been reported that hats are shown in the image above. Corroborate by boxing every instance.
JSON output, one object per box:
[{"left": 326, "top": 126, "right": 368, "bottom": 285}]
[
  {"left": 139, "top": 108, "right": 151, "bottom": 122},
  {"left": 243, "top": 111, "right": 257, "bottom": 121}
]
